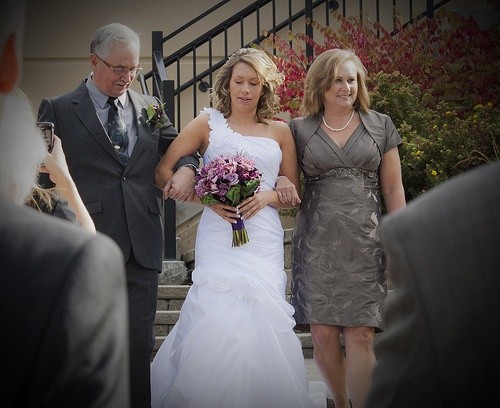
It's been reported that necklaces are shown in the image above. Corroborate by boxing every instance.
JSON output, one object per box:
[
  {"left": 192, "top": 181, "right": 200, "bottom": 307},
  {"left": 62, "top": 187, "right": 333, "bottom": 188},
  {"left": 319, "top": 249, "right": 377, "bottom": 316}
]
[{"left": 323, "top": 110, "right": 355, "bottom": 131}]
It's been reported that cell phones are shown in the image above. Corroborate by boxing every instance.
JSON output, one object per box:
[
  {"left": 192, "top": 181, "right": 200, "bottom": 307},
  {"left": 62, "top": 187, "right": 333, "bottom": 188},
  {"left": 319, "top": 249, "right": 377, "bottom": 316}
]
[{"left": 35, "top": 122, "right": 54, "bottom": 168}]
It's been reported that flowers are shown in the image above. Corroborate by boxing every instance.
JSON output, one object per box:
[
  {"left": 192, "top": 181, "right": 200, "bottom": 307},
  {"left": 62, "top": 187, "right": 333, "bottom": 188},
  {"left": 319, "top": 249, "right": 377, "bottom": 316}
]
[
  {"left": 147, "top": 100, "right": 166, "bottom": 126},
  {"left": 192, "top": 152, "right": 263, "bottom": 248}
]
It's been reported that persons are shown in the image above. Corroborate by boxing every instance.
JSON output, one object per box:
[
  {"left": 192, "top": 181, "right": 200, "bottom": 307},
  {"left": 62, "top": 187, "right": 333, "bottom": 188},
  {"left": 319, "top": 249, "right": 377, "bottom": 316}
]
[
  {"left": 276, "top": 47, "right": 406, "bottom": 408},
  {"left": 367, "top": 158, "right": 500, "bottom": 408},
  {"left": 0, "top": 0, "right": 131, "bottom": 408},
  {"left": 149, "top": 48, "right": 325, "bottom": 408},
  {"left": 37, "top": 23, "right": 201, "bottom": 408},
  {"left": 0, "top": 87, "right": 96, "bottom": 232}
]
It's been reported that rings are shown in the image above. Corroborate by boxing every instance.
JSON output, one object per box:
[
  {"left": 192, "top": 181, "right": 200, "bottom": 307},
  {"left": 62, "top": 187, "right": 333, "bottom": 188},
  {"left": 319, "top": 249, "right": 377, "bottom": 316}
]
[{"left": 282, "top": 193, "right": 287, "bottom": 196}]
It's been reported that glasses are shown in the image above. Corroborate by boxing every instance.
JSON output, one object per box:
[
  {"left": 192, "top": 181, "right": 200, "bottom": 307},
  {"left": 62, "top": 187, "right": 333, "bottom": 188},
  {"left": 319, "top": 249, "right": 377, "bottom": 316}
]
[{"left": 95, "top": 53, "right": 142, "bottom": 78}]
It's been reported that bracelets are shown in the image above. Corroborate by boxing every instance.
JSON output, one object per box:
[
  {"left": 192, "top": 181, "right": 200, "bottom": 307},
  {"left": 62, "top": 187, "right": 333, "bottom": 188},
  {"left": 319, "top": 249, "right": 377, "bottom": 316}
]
[{"left": 277, "top": 175, "right": 287, "bottom": 179}]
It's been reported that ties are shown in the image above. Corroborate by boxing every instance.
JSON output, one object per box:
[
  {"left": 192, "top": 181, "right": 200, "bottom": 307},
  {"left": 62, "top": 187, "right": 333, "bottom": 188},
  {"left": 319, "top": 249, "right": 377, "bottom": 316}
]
[{"left": 106, "top": 96, "right": 128, "bottom": 162}]
